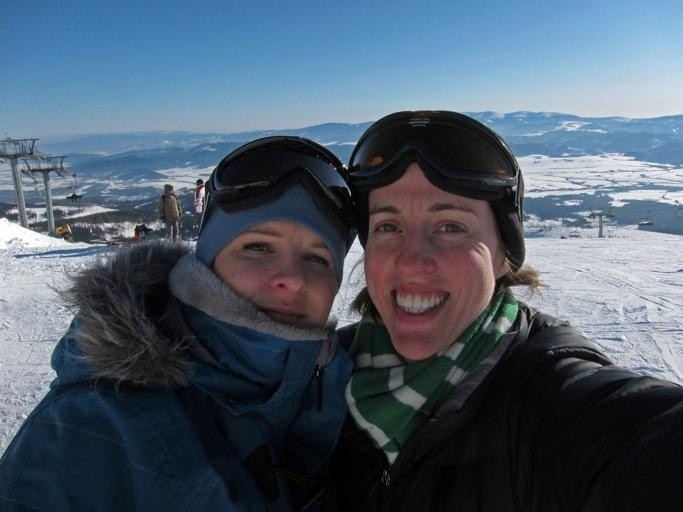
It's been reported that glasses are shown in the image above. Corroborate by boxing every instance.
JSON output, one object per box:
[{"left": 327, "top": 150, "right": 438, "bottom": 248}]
[
  {"left": 198, "top": 135, "right": 360, "bottom": 246},
  {"left": 346, "top": 109, "right": 525, "bottom": 223}
]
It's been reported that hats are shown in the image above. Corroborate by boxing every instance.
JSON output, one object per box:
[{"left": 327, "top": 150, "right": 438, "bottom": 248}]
[{"left": 195, "top": 189, "right": 345, "bottom": 294}]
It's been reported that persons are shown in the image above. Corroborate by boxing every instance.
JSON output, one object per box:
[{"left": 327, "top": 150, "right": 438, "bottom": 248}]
[
  {"left": 0, "top": 134, "right": 360, "bottom": 511},
  {"left": 343, "top": 108, "right": 683, "bottom": 511},
  {"left": 193, "top": 178, "right": 207, "bottom": 221},
  {"left": 158, "top": 183, "right": 182, "bottom": 241}
]
